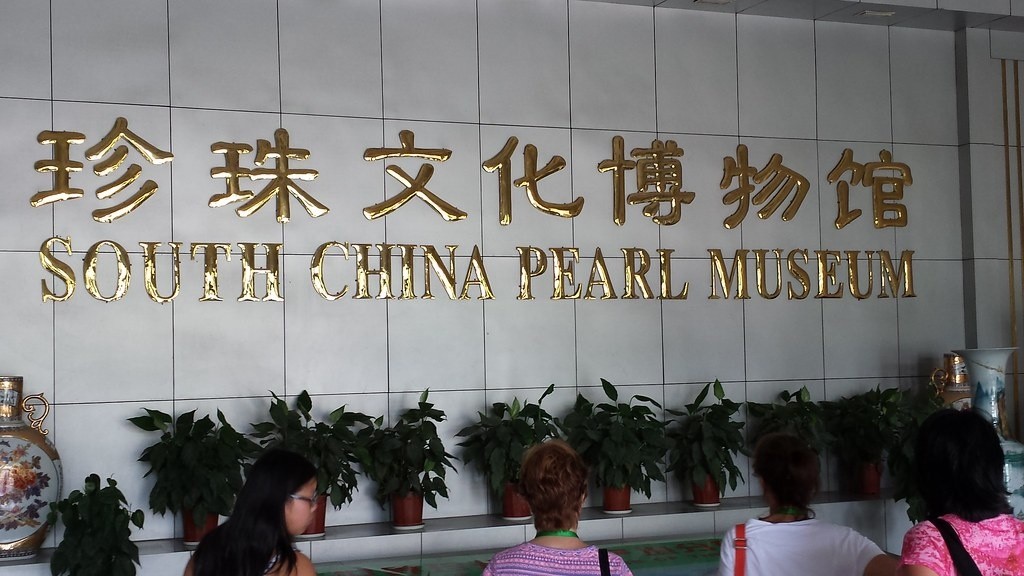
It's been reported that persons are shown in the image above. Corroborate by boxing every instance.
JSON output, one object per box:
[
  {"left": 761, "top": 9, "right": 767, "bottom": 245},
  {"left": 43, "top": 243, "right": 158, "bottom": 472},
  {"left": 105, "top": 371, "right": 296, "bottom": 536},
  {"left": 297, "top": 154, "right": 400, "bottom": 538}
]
[
  {"left": 898, "top": 406, "right": 1024, "bottom": 576},
  {"left": 720, "top": 432, "right": 900, "bottom": 576},
  {"left": 482, "top": 441, "right": 636, "bottom": 576},
  {"left": 184, "top": 443, "right": 318, "bottom": 576}
]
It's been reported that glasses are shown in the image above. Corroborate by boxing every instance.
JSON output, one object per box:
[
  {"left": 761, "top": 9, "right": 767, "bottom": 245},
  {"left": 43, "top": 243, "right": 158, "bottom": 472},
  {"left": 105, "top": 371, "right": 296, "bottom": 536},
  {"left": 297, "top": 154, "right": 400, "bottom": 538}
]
[{"left": 287, "top": 491, "right": 318, "bottom": 509}]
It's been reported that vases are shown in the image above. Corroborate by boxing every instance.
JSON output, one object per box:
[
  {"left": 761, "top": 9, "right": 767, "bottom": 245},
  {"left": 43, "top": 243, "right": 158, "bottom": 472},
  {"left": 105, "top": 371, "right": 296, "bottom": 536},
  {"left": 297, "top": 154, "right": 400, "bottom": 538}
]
[
  {"left": 931, "top": 352, "right": 972, "bottom": 410},
  {"left": 951, "top": 346, "right": 1024, "bottom": 520},
  {"left": 0, "top": 376, "right": 63, "bottom": 563}
]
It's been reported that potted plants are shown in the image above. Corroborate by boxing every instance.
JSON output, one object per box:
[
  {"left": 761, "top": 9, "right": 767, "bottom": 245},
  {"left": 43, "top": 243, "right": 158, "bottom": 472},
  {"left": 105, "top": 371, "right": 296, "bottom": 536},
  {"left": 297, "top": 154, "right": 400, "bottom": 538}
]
[
  {"left": 364, "top": 387, "right": 462, "bottom": 527},
  {"left": 452, "top": 383, "right": 571, "bottom": 518},
  {"left": 821, "top": 383, "right": 916, "bottom": 497},
  {"left": 565, "top": 378, "right": 679, "bottom": 510},
  {"left": 247, "top": 389, "right": 375, "bottom": 535},
  {"left": 125, "top": 407, "right": 265, "bottom": 543},
  {"left": 747, "top": 385, "right": 839, "bottom": 498},
  {"left": 661, "top": 378, "right": 755, "bottom": 505}
]
